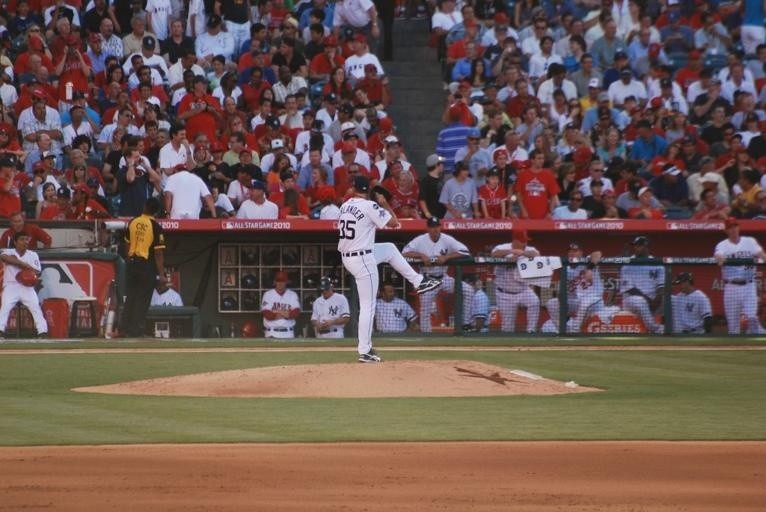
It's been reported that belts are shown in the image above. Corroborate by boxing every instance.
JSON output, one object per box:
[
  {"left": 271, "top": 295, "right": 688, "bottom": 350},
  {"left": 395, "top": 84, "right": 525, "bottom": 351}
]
[
  {"left": 319, "top": 328, "right": 338, "bottom": 333},
  {"left": 428, "top": 274, "right": 444, "bottom": 279},
  {"left": 264, "top": 327, "right": 293, "bottom": 332},
  {"left": 497, "top": 287, "right": 521, "bottom": 294},
  {"left": 682, "top": 328, "right": 696, "bottom": 333},
  {"left": 724, "top": 279, "right": 753, "bottom": 285},
  {"left": 342, "top": 249, "right": 372, "bottom": 257}
]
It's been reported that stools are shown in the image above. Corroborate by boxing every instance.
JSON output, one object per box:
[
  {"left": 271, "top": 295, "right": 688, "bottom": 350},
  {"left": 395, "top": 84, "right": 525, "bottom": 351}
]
[
  {"left": 14, "top": 302, "right": 29, "bottom": 338},
  {"left": 69, "top": 297, "right": 99, "bottom": 336}
]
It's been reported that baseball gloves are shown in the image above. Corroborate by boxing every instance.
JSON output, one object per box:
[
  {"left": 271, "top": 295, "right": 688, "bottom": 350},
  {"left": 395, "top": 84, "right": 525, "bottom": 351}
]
[{"left": 370, "top": 185, "right": 392, "bottom": 204}]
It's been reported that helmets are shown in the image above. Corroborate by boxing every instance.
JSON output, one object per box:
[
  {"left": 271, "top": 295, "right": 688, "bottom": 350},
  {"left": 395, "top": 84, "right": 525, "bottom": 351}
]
[
  {"left": 16, "top": 270, "right": 38, "bottom": 286},
  {"left": 240, "top": 319, "right": 262, "bottom": 338},
  {"left": 221, "top": 252, "right": 355, "bottom": 312}
]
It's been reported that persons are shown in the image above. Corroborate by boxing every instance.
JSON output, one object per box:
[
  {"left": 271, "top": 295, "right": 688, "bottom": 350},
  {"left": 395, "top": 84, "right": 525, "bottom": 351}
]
[
  {"left": 309, "top": 276, "right": 351, "bottom": 338},
  {"left": 491, "top": 230, "right": 539, "bottom": 333},
  {"left": 0, "top": 210, "right": 53, "bottom": 250},
  {"left": 150, "top": 271, "right": 183, "bottom": 338},
  {"left": 374, "top": 282, "right": 419, "bottom": 334},
  {"left": 673, "top": 270, "right": 713, "bottom": 336},
  {"left": 546, "top": 241, "right": 604, "bottom": 334},
  {"left": 401, "top": 217, "right": 471, "bottom": 334},
  {"left": 336, "top": 175, "right": 443, "bottom": 362},
  {"left": 260, "top": 271, "right": 300, "bottom": 338},
  {"left": 0, "top": 232, "right": 51, "bottom": 342},
  {"left": 467, "top": 275, "right": 491, "bottom": 333},
  {"left": 621, "top": 234, "right": 666, "bottom": 334},
  {"left": 713, "top": 217, "right": 766, "bottom": 335},
  {"left": 109, "top": 198, "right": 168, "bottom": 337},
  {"left": 0, "top": 0, "right": 423, "bottom": 219},
  {"left": 418, "top": 0, "right": 765, "bottom": 221}
]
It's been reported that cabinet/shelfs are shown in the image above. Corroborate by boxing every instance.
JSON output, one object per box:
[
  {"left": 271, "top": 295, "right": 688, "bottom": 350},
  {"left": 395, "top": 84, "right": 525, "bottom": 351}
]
[{"left": 218, "top": 240, "right": 407, "bottom": 315}]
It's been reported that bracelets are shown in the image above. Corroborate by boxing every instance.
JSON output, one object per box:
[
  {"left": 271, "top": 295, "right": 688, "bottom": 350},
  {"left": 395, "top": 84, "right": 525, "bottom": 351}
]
[{"left": 586, "top": 261, "right": 594, "bottom": 269}]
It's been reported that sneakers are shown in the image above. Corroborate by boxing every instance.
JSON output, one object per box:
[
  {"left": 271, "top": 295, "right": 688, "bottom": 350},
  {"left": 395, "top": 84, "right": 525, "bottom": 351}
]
[
  {"left": 0, "top": 329, "right": 6, "bottom": 339},
  {"left": 358, "top": 348, "right": 382, "bottom": 363},
  {"left": 416, "top": 279, "right": 443, "bottom": 294},
  {"left": 121, "top": 328, "right": 153, "bottom": 338},
  {"left": 37, "top": 332, "right": 49, "bottom": 339}
]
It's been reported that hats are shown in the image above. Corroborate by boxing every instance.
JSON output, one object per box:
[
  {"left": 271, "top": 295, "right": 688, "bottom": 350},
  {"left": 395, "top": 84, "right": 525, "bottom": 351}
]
[
  {"left": 155, "top": 273, "right": 166, "bottom": 286},
  {"left": 725, "top": 217, "right": 740, "bottom": 228},
  {"left": 272, "top": 271, "right": 289, "bottom": 285},
  {"left": 427, "top": 216, "right": 442, "bottom": 227},
  {"left": 425, "top": 154, "right": 448, "bottom": 168},
  {"left": 460, "top": 0, "right": 758, "bottom": 198},
  {"left": 566, "top": 241, "right": 583, "bottom": 251},
  {"left": 318, "top": 276, "right": 332, "bottom": 292},
  {"left": 671, "top": 272, "right": 691, "bottom": 286},
  {"left": 512, "top": 227, "right": 533, "bottom": 242},
  {"left": 630, "top": 236, "right": 651, "bottom": 249},
  {"left": 0, "top": 13, "right": 403, "bottom": 198}
]
